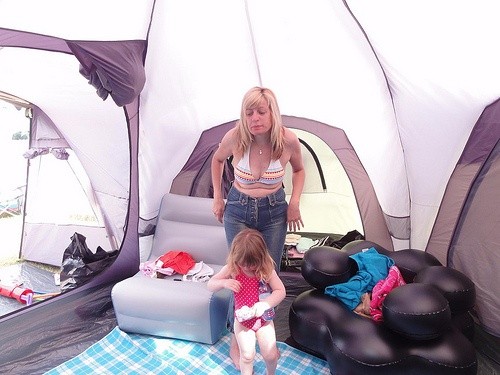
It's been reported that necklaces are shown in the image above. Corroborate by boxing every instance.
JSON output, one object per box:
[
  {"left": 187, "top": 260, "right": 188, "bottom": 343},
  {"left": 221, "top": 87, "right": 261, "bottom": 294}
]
[{"left": 252, "top": 136, "right": 267, "bottom": 155}]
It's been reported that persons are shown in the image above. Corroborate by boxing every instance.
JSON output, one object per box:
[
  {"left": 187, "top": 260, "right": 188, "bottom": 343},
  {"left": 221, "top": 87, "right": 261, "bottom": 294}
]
[
  {"left": 209, "top": 229, "right": 286, "bottom": 375},
  {"left": 211, "top": 86, "right": 306, "bottom": 371}
]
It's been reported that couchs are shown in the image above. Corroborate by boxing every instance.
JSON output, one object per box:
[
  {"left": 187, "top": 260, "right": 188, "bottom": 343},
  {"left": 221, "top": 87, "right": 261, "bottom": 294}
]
[
  {"left": 289, "top": 238, "right": 479, "bottom": 375},
  {"left": 110, "top": 193, "right": 232, "bottom": 345}
]
